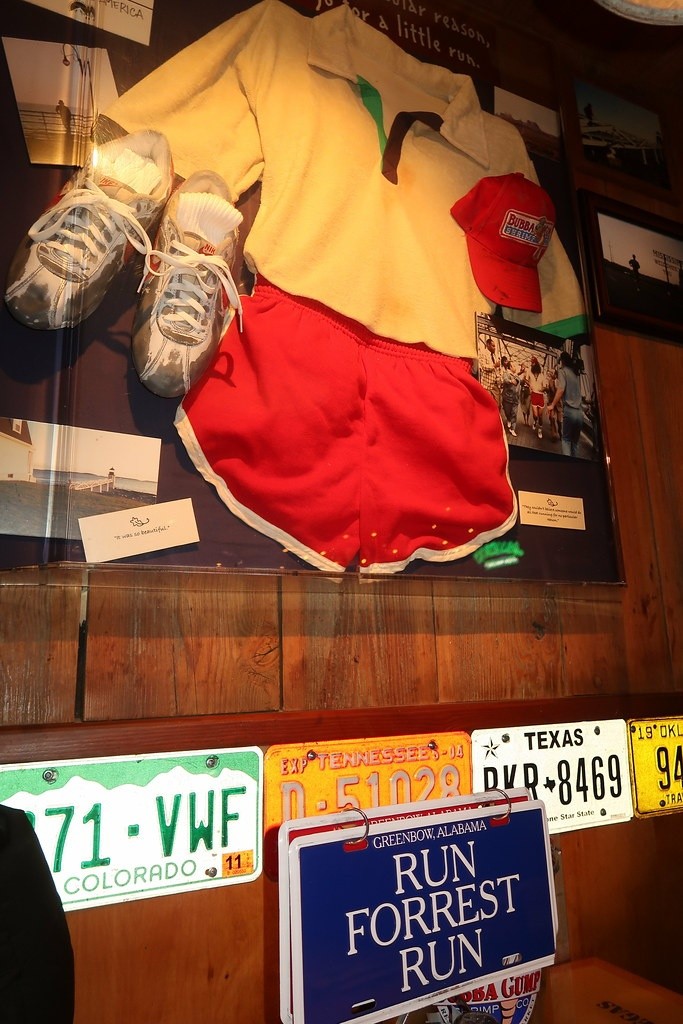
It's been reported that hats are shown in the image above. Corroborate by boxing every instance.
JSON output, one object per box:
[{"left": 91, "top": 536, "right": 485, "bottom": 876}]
[
  {"left": 453, "top": 171, "right": 555, "bottom": 313},
  {"left": 529, "top": 357, "right": 539, "bottom": 363}
]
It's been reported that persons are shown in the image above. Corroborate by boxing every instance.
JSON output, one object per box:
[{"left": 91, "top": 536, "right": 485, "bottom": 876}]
[
  {"left": 525, "top": 357, "right": 546, "bottom": 438},
  {"left": 590, "top": 382, "right": 603, "bottom": 461},
  {"left": 629, "top": 253, "right": 641, "bottom": 291},
  {"left": 55, "top": 100, "right": 72, "bottom": 134},
  {"left": 478, "top": 339, "right": 502, "bottom": 412},
  {"left": 495, "top": 356, "right": 565, "bottom": 442},
  {"left": 547, "top": 351, "right": 584, "bottom": 458}
]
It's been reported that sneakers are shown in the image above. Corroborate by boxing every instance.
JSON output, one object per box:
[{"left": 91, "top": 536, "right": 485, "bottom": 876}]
[
  {"left": 538, "top": 429, "right": 543, "bottom": 439},
  {"left": 1, "top": 132, "right": 176, "bottom": 331},
  {"left": 533, "top": 422, "right": 537, "bottom": 431},
  {"left": 509, "top": 429, "right": 517, "bottom": 437},
  {"left": 507, "top": 420, "right": 512, "bottom": 428},
  {"left": 134, "top": 167, "right": 243, "bottom": 399}
]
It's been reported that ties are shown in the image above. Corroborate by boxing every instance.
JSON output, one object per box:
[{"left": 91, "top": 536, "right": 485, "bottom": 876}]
[{"left": 491, "top": 353, "right": 495, "bottom": 364}]
